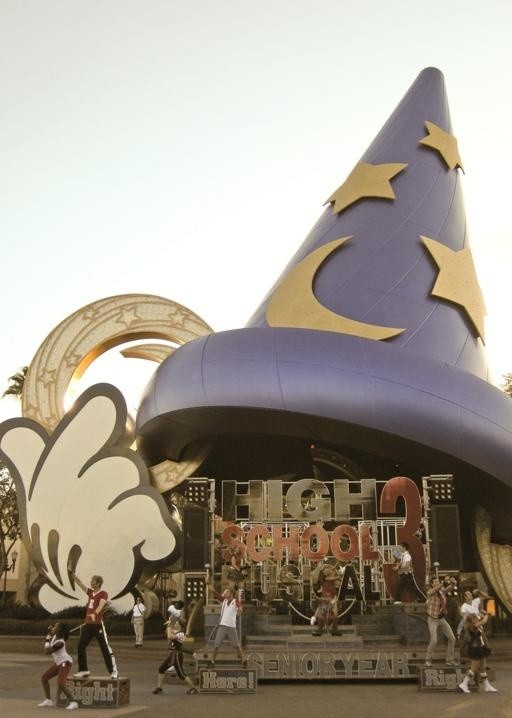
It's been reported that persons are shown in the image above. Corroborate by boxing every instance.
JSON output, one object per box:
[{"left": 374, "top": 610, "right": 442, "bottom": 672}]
[
  {"left": 67, "top": 559, "right": 118, "bottom": 679},
  {"left": 459, "top": 609, "right": 498, "bottom": 693},
  {"left": 152, "top": 619, "right": 200, "bottom": 694},
  {"left": 312, "top": 567, "right": 343, "bottom": 636},
  {"left": 391, "top": 542, "right": 429, "bottom": 603},
  {"left": 130, "top": 597, "right": 146, "bottom": 648},
  {"left": 426, "top": 576, "right": 459, "bottom": 667},
  {"left": 206, "top": 581, "right": 248, "bottom": 668},
  {"left": 38, "top": 621, "right": 79, "bottom": 710},
  {"left": 163, "top": 591, "right": 186, "bottom": 672},
  {"left": 223, "top": 543, "right": 244, "bottom": 605},
  {"left": 456, "top": 589, "right": 488, "bottom": 641}
]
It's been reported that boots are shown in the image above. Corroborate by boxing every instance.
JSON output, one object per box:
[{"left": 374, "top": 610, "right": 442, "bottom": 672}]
[
  {"left": 480, "top": 672, "right": 497, "bottom": 692},
  {"left": 458, "top": 670, "right": 475, "bottom": 693}
]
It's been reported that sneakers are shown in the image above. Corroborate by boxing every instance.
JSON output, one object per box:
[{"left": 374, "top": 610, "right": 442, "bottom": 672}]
[
  {"left": 153, "top": 688, "right": 163, "bottom": 694},
  {"left": 446, "top": 660, "right": 457, "bottom": 666},
  {"left": 186, "top": 688, "right": 198, "bottom": 695},
  {"left": 65, "top": 702, "right": 78, "bottom": 709},
  {"left": 38, "top": 699, "right": 53, "bottom": 708},
  {"left": 207, "top": 662, "right": 214, "bottom": 668},
  {"left": 110, "top": 671, "right": 118, "bottom": 679},
  {"left": 425, "top": 660, "right": 432, "bottom": 666},
  {"left": 242, "top": 658, "right": 247, "bottom": 665},
  {"left": 393, "top": 600, "right": 402, "bottom": 605},
  {"left": 73, "top": 671, "right": 91, "bottom": 678}
]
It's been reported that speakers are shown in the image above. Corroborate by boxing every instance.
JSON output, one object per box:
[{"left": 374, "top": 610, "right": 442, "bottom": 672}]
[
  {"left": 181, "top": 507, "right": 210, "bottom": 572},
  {"left": 430, "top": 502, "right": 464, "bottom": 571}
]
